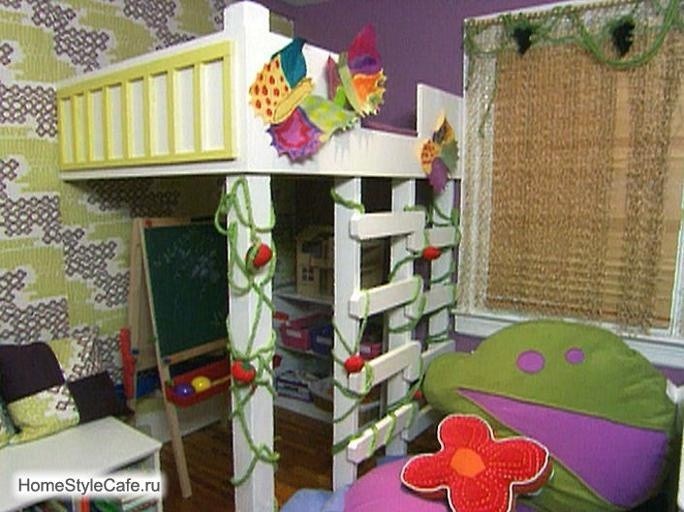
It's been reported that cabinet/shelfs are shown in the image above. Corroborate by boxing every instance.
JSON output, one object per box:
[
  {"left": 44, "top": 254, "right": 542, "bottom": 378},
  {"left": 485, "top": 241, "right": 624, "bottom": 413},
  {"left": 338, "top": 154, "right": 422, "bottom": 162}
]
[
  {"left": 0, "top": 418, "right": 164, "bottom": 512},
  {"left": 273, "top": 288, "right": 387, "bottom": 453}
]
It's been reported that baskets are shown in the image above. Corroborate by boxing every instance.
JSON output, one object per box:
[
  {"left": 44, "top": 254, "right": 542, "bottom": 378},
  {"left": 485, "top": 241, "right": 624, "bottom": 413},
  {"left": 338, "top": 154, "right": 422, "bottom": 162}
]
[{"left": 310, "top": 376, "right": 379, "bottom": 426}]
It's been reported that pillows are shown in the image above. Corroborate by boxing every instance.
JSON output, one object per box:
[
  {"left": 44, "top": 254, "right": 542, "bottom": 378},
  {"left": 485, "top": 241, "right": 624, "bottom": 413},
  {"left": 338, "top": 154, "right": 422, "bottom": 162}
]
[{"left": 1, "top": 334, "right": 133, "bottom": 444}]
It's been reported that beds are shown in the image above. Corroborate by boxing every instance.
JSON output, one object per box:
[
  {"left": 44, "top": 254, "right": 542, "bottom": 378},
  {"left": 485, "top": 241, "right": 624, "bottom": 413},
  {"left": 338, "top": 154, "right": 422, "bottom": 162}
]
[{"left": 56, "top": 3, "right": 462, "bottom": 512}]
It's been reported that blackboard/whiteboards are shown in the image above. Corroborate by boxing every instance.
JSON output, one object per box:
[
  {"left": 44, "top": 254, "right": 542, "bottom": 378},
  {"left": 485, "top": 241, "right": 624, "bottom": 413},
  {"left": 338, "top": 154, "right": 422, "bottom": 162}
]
[{"left": 138, "top": 216, "right": 230, "bottom": 366}]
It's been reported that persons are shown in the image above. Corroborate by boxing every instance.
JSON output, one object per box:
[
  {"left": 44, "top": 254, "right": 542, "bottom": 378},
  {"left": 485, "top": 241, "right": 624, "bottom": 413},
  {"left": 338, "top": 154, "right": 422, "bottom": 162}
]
[
  {"left": 191, "top": 376, "right": 211, "bottom": 393},
  {"left": 176, "top": 384, "right": 192, "bottom": 397}
]
